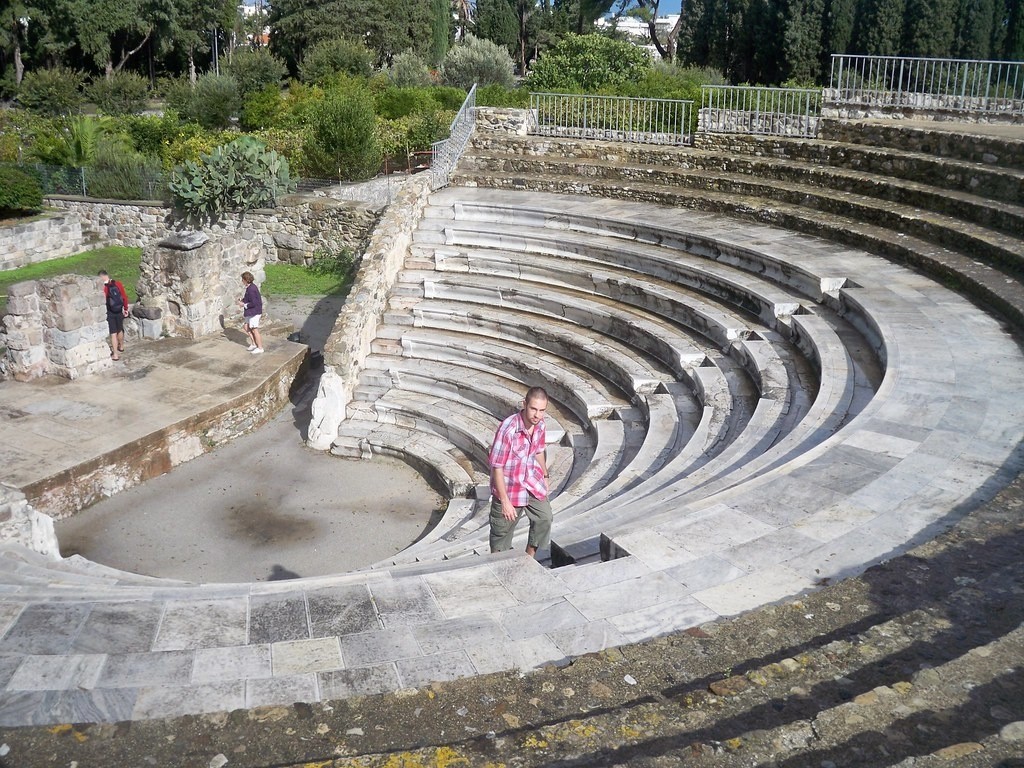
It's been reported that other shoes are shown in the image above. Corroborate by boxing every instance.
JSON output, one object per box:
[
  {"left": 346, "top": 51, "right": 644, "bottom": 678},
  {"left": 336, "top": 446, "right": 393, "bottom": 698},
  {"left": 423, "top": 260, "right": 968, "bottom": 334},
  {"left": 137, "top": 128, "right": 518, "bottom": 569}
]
[
  {"left": 247, "top": 345, "right": 257, "bottom": 351},
  {"left": 250, "top": 348, "right": 264, "bottom": 354}
]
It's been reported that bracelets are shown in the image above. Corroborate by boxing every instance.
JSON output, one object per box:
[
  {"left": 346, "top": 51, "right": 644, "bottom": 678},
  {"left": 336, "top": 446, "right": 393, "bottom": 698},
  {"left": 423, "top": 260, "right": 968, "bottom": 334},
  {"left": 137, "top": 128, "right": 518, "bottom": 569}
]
[{"left": 543, "top": 474, "right": 549, "bottom": 478}]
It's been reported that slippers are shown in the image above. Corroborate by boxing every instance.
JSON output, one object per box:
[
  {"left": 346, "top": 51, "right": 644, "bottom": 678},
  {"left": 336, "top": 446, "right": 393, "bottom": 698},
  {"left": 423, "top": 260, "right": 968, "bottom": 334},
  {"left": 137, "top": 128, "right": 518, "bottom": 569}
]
[
  {"left": 118, "top": 343, "right": 124, "bottom": 352},
  {"left": 111, "top": 354, "right": 119, "bottom": 361}
]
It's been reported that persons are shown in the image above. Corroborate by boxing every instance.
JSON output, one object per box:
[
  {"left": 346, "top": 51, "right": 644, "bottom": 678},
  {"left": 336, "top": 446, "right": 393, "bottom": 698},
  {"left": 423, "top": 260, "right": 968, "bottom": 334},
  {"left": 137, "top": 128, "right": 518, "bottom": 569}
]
[
  {"left": 98, "top": 269, "right": 129, "bottom": 361},
  {"left": 488, "top": 387, "right": 554, "bottom": 559},
  {"left": 235, "top": 272, "right": 264, "bottom": 354}
]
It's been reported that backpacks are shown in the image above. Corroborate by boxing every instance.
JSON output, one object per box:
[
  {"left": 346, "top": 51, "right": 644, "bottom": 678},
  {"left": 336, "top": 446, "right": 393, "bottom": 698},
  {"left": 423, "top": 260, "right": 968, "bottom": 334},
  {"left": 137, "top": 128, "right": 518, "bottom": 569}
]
[{"left": 104, "top": 279, "right": 124, "bottom": 314}]
